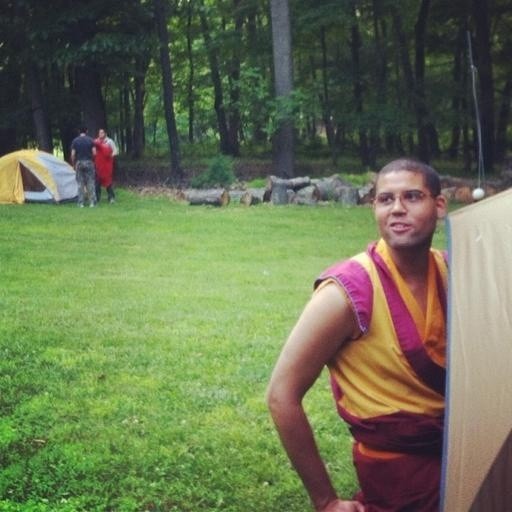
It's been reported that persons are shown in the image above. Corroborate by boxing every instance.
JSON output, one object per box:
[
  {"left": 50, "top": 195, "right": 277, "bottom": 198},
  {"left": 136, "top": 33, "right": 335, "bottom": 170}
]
[
  {"left": 266, "top": 160, "right": 448, "bottom": 511},
  {"left": 93, "top": 129, "right": 120, "bottom": 204},
  {"left": 70, "top": 125, "right": 98, "bottom": 207}
]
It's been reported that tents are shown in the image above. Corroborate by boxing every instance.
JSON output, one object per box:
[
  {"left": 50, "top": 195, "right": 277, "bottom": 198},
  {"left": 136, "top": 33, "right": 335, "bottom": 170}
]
[{"left": 1, "top": 149, "right": 88, "bottom": 206}]
